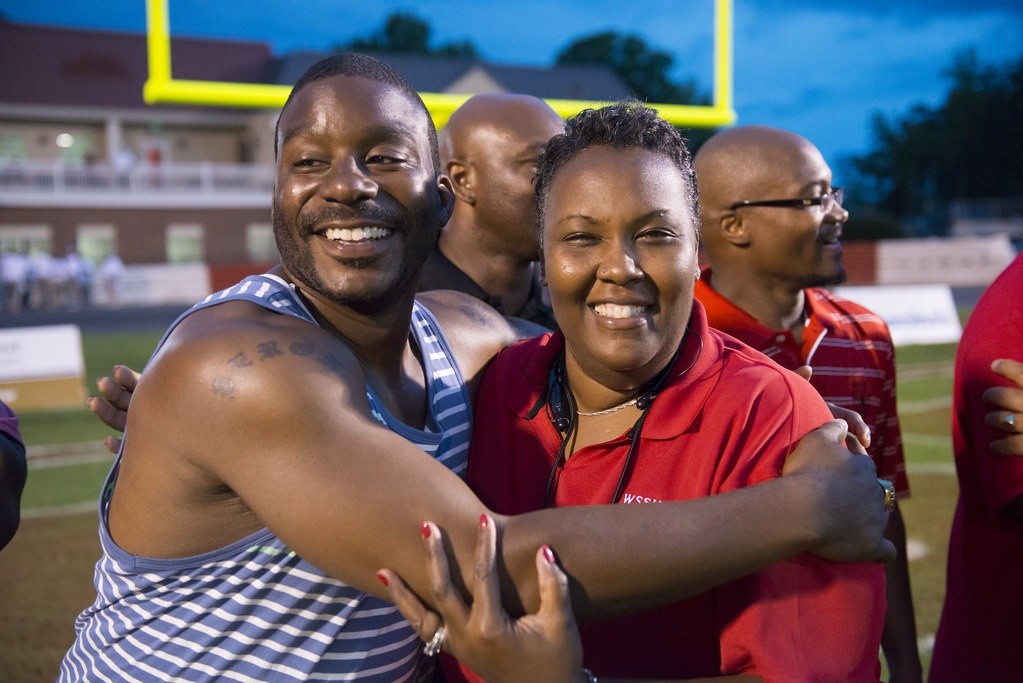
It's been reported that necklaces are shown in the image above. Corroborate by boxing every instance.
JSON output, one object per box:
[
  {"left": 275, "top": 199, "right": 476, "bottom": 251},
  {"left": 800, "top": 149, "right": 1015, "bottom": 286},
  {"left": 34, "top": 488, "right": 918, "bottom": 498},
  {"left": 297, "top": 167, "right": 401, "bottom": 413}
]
[{"left": 568, "top": 385, "right": 646, "bottom": 417}]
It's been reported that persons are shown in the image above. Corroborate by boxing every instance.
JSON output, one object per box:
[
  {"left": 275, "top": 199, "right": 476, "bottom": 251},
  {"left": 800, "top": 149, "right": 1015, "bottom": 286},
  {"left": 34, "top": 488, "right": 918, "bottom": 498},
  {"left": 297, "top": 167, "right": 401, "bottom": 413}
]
[{"left": 1, "top": 48, "right": 1022, "bottom": 683}]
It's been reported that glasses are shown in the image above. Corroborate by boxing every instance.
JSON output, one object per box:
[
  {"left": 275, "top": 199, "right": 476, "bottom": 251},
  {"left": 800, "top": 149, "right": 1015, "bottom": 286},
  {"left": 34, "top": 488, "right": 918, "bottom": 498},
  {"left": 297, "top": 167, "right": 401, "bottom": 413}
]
[{"left": 730, "top": 187, "right": 844, "bottom": 213}]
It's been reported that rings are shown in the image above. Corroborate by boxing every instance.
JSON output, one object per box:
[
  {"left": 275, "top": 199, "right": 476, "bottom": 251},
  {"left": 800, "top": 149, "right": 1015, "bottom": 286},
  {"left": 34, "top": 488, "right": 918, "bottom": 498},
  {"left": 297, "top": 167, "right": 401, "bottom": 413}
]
[
  {"left": 1001, "top": 412, "right": 1016, "bottom": 435},
  {"left": 423, "top": 626, "right": 445, "bottom": 657},
  {"left": 877, "top": 477, "right": 896, "bottom": 508}
]
